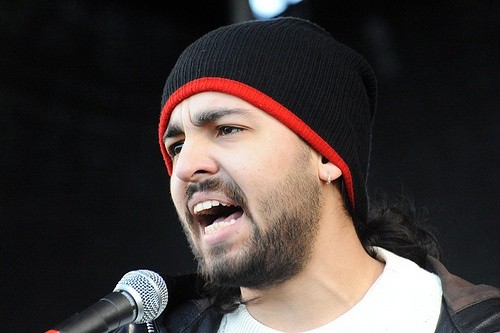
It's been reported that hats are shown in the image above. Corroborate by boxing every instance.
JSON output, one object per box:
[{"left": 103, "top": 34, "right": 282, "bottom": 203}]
[{"left": 158, "top": 17, "right": 378, "bottom": 224}]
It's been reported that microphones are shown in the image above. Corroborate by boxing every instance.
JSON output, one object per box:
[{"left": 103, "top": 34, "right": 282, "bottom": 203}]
[{"left": 44, "top": 269, "right": 168, "bottom": 333}]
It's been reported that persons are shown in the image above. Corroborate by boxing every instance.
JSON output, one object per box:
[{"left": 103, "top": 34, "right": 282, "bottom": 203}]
[{"left": 96, "top": 18, "right": 500, "bottom": 333}]
